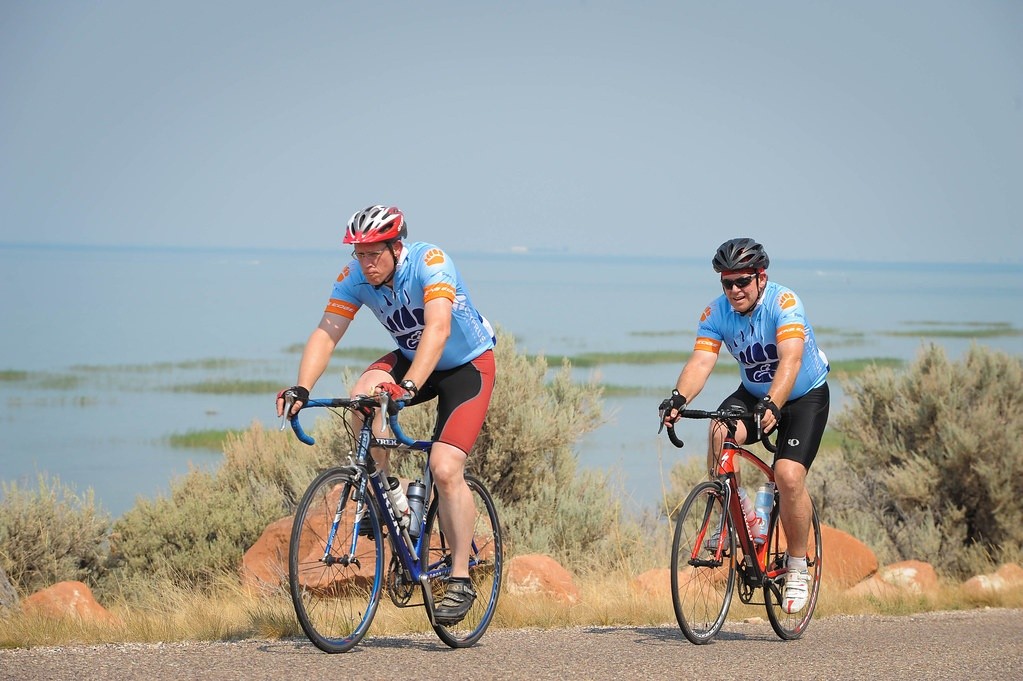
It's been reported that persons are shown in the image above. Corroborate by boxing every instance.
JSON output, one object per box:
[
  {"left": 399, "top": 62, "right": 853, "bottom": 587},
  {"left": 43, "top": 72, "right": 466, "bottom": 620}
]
[
  {"left": 275, "top": 206, "right": 495, "bottom": 625},
  {"left": 658, "top": 238, "right": 830, "bottom": 614}
]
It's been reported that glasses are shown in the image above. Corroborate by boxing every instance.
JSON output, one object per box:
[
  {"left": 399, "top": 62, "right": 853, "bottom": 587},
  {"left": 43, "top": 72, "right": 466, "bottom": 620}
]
[
  {"left": 720, "top": 273, "right": 760, "bottom": 291},
  {"left": 350, "top": 248, "right": 390, "bottom": 262}
]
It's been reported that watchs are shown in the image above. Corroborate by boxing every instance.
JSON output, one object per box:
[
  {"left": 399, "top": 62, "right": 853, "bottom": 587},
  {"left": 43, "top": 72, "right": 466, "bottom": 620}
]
[{"left": 398, "top": 380, "right": 418, "bottom": 397}]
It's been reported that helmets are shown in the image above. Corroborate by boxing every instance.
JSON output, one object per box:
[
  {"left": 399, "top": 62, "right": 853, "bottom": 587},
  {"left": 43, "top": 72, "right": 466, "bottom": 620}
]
[
  {"left": 715, "top": 239, "right": 769, "bottom": 275},
  {"left": 341, "top": 205, "right": 408, "bottom": 243}
]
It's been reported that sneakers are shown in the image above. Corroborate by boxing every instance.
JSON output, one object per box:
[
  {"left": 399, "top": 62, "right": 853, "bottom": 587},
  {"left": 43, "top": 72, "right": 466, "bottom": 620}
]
[
  {"left": 704, "top": 516, "right": 747, "bottom": 553},
  {"left": 355, "top": 496, "right": 389, "bottom": 535},
  {"left": 780, "top": 558, "right": 814, "bottom": 615},
  {"left": 431, "top": 574, "right": 477, "bottom": 625}
]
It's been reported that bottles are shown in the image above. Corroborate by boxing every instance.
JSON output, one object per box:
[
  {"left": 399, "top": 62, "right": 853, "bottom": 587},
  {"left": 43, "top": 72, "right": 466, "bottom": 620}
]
[
  {"left": 737, "top": 486, "right": 760, "bottom": 539},
  {"left": 406, "top": 478, "right": 426, "bottom": 537},
  {"left": 754, "top": 481, "right": 775, "bottom": 545},
  {"left": 387, "top": 477, "right": 412, "bottom": 532}
]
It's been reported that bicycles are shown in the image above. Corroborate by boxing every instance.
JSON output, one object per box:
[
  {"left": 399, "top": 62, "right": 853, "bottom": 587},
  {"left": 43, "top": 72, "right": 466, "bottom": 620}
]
[
  {"left": 664, "top": 404, "right": 823, "bottom": 646},
  {"left": 288, "top": 396, "right": 507, "bottom": 654}
]
